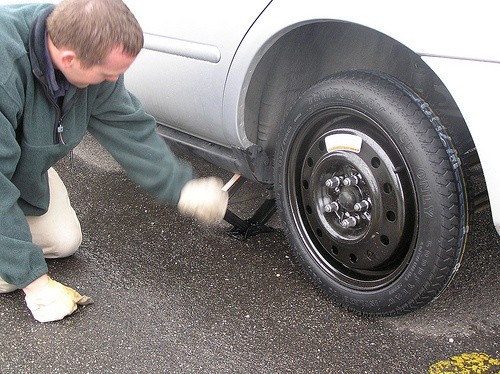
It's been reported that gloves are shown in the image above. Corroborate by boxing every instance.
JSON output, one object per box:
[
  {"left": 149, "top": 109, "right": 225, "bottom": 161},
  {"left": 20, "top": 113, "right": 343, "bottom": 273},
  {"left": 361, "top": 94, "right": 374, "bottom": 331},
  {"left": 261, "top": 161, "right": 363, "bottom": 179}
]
[
  {"left": 179, "top": 176, "right": 229, "bottom": 228},
  {"left": 22, "top": 274, "right": 93, "bottom": 322}
]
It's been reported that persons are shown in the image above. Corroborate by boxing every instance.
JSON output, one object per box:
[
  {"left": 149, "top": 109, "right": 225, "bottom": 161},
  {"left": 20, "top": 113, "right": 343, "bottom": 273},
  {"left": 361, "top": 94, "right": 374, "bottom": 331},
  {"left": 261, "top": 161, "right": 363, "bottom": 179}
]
[{"left": 0, "top": 0, "right": 228, "bottom": 321}]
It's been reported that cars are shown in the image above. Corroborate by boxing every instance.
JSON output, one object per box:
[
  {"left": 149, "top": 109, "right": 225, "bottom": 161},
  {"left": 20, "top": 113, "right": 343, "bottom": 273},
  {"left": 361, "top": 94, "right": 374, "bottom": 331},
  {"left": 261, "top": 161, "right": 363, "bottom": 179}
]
[{"left": 123, "top": 1, "right": 500, "bottom": 316}]
